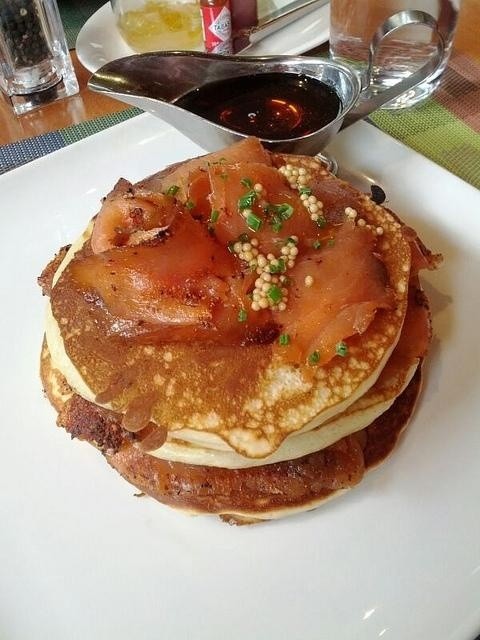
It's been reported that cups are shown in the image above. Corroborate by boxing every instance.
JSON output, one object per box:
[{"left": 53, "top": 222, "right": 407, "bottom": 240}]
[
  {"left": 109, "top": 1, "right": 205, "bottom": 52},
  {"left": 2, "top": 0, "right": 80, "bottom": 118},
  {"left": 328, "top": 0, "right": 459, "bottom": 111}
]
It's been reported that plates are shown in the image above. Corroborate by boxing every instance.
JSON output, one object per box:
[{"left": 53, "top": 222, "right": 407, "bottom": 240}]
[
  {"left": 0, "top": 81, "right": 479, "bottom": 640},
  {"left": 74, "top": 1, "right": 460, "bottom": 75}
]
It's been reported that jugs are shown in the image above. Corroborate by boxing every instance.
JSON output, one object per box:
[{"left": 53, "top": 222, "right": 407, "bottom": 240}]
[{"left": 86, "top": 50, "right": 363, "bottom": 175}]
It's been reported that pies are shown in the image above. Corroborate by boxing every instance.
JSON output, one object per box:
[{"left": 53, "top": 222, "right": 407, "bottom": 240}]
[{"left": 41, "top": 152, "right": 433, "bottom": 521}]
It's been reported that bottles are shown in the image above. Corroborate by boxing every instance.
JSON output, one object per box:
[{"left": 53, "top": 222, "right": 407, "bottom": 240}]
[{"left": 200, "top": 0, "right": 235, "bottom": 57}]
